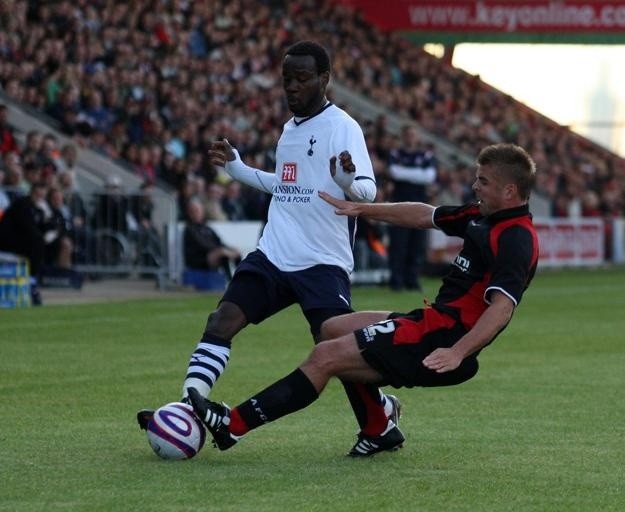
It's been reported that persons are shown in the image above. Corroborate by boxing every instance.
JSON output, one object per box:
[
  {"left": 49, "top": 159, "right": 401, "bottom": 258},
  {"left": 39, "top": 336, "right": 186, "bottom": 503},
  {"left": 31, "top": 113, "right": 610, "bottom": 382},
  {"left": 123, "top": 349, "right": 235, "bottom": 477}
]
[
  {"left": 136, "top": 40, "right": 407, "bottom": 453},
  {"left": 185, "top": 140, "right": 540, "bottom": 461}
]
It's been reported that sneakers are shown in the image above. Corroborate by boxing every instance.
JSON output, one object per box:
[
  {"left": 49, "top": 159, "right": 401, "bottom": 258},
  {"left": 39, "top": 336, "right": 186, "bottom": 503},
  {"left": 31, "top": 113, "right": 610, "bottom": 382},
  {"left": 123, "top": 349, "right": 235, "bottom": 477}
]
[
  {"left": 385, "top": 394, "right": 401, "bottom": 427},
  {"left": 344, "top": 419, "right": 405, "bottom": 459},
  {"left": 187, "top": 386, "right": 245, "bottom": 452},
  {"left": 137, "top": 409, "right": 158, "bottom": 434}
]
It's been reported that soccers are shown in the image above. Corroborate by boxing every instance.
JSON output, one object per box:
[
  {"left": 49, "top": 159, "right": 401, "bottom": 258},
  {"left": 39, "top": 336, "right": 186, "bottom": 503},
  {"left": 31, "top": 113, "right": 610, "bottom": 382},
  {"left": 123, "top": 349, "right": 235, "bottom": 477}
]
[{"left": 146, "top": 401, "right": 206, "bottom": 461}]
